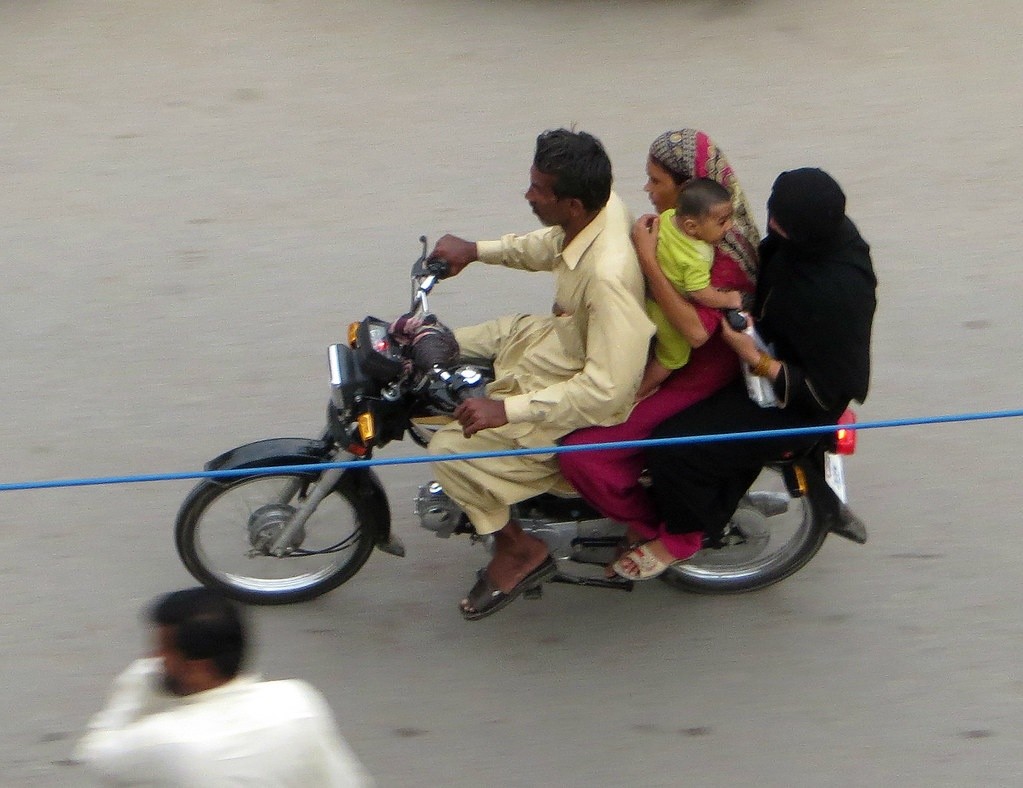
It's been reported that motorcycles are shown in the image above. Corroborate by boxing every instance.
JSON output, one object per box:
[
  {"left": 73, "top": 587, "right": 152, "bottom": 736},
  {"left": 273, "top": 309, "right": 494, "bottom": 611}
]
[{"left": 171, "top": 234, "right": 869, "bottom": 605}]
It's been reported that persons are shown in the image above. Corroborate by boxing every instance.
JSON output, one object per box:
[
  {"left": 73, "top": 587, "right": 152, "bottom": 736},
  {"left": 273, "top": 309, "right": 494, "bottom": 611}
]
[
  {"left": 557, "top": 129, "right": 760, "bottom": 581},
  {"left": 72, "top": 589, "right": 374, "bottom": 788},
  {"left": 428, "top": 129, "right": 656, "bottom": 623},
  {"left": 645, "top": 168, "right": 878, "bottom": 546},
  {"left": 637, "top": 177, "right": 743, "bottom": 399}
]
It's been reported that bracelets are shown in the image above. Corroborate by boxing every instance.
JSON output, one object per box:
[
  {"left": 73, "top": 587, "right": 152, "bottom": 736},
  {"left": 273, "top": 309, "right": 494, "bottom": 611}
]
[{"left": 749, "top": 350, "right": 771, "bottom": 377}]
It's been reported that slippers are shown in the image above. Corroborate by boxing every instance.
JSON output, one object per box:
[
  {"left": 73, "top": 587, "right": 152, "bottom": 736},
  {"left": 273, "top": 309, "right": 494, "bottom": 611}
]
[
  {"left": 612, "top": 542, "right": 701, "bottom": 581},
  {"left": 459, "top": 553, "right": 559, "bottom": 621}
]
